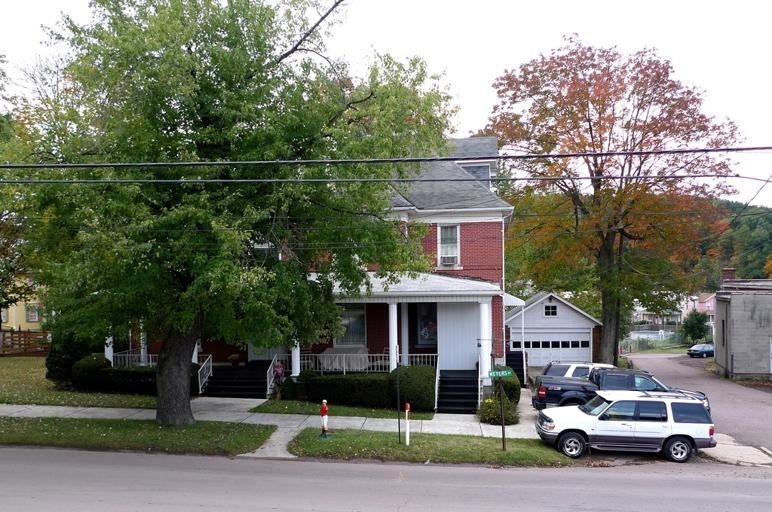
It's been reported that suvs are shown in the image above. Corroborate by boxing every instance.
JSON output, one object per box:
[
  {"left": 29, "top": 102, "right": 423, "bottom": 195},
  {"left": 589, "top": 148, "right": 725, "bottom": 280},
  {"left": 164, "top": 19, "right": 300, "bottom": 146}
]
[{"left": 529, "top": 358, "right": 722, "bottom": 465}]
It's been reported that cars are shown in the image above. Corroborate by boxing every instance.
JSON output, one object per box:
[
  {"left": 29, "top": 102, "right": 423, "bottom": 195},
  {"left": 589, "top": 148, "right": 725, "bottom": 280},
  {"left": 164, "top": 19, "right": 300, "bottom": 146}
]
[{"left": 686, "top": 343, "right": 715, "bottom": 360}]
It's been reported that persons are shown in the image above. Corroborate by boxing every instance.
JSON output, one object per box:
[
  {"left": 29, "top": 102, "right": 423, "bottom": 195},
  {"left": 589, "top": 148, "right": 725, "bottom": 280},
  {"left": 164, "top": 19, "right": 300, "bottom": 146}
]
[
  {"left": 318, "top": 399, "right": 329, "bottom": 439},
  {"left": 272, "top": 362, "right": 285, "bottom": 384}
]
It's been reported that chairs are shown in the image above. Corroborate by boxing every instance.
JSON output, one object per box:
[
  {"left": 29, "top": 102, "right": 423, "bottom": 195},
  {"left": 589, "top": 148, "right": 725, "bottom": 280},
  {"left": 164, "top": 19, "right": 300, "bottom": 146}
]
[
  {"left": 372, "top": 348, "right": 389, "bottom": 366},
  {"left": 301, "top": 361, "right": 313, "bottom": 370}
]
